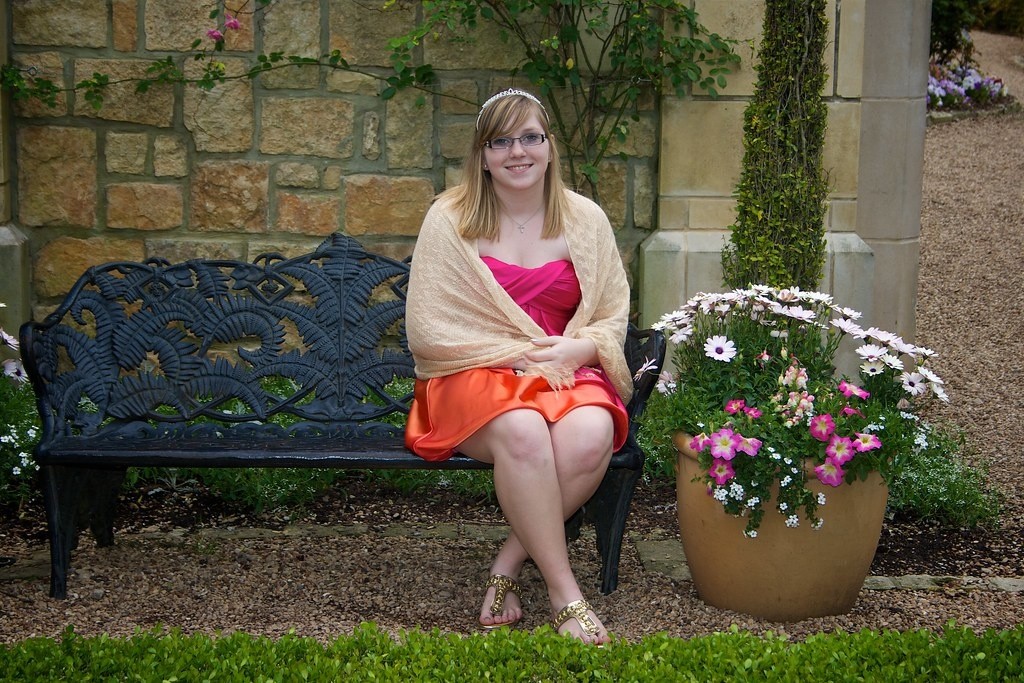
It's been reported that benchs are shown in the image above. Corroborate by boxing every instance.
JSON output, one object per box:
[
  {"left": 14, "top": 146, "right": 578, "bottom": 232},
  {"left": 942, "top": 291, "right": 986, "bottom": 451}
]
[{"left": 19, "top": 233, "right": 667, "bottom": 600}]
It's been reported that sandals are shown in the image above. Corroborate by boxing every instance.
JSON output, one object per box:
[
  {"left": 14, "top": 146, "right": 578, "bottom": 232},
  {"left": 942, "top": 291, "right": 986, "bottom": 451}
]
[
  {"left": 476, "top": 574, "right": 524, "bottom": 630},
  {"left": 552, "top": 599, "right": 616, "bottom": 648}
]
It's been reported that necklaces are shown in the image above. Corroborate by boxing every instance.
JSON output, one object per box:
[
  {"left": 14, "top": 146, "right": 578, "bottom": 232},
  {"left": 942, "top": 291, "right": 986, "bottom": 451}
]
[{"left": 497, "top": 198, "right": 545, "bottom": 234}]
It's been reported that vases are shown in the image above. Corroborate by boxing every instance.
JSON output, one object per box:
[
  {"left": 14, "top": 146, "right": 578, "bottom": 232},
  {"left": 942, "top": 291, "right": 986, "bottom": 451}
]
[{"left": 667, "top": 428, "right": 892, "bottom": 625}]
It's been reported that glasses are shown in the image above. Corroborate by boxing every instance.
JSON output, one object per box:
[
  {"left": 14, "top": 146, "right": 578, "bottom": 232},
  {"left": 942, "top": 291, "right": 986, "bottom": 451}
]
[{"left": 484, "top": 134, "right": 548, "bottom": 149}]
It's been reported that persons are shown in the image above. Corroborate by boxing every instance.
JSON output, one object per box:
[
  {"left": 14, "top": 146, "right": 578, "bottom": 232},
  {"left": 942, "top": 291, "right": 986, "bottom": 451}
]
[{"left": 402, "top": 86, "right": 633, "bottom": 650}]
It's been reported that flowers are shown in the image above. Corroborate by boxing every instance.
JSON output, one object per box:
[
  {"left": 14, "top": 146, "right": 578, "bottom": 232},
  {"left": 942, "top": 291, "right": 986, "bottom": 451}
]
[
  {"left": 0, "top": 302, "right": 40, "bottom": 475},
  {"left": 206, "top": 12, "right": 241, "bottom": 40},
  {"left": 928, "top": 61, "right": 1008, "bottom": 107},
  {"left": 634, "top": 283, "right": 951, "bottom": 537}
]
